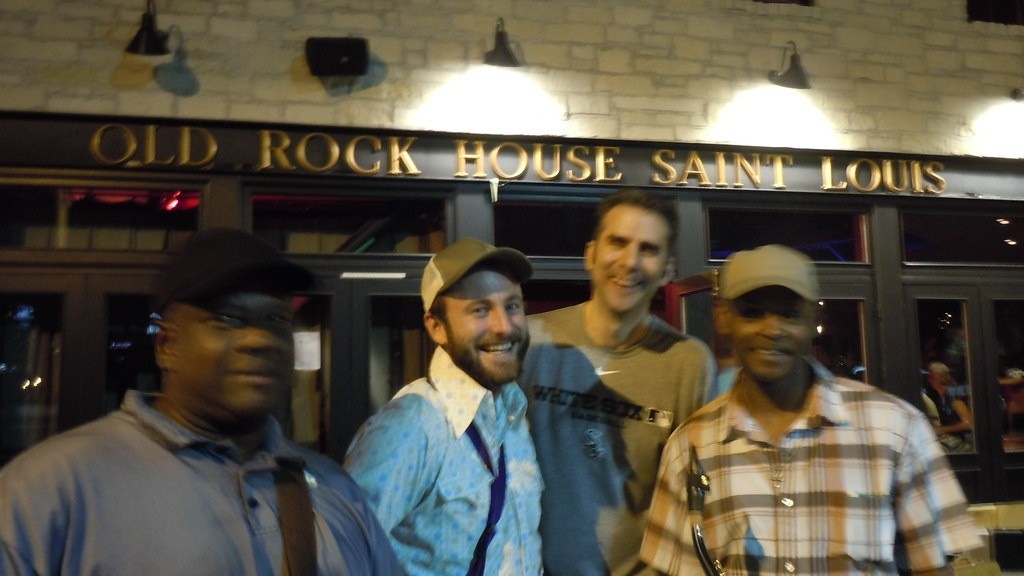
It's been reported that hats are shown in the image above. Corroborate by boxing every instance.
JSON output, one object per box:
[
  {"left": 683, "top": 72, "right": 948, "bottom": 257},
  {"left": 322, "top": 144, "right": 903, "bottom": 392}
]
[
  {"left": 421, "top": 236, "right": 534, "bottom": 313},
  {"left": 148, "top": 226, "right": 316, "bottom": 309},
  {"left": 719, "top": 243, "right": 818, "bottom": 303}
]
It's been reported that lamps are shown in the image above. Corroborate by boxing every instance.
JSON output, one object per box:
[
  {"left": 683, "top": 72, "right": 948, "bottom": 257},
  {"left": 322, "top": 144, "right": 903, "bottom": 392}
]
[
  {"left": 477, "top": 16, "right": 520, "bottom": 68},
  {"left": 125, "top": 1, "right": 173, "bottom": 56},
  {"left": 766, "top": 41, "right": 812, "bottom": 90}
]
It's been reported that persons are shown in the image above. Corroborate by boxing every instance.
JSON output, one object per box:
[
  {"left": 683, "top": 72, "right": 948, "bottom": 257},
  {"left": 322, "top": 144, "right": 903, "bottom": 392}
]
[
  {"left": 0, "top": 226, "right": 408, "bottom": 576},
  {"left": 342, "top": 190, "right": 985, "bottom": 576}
]
